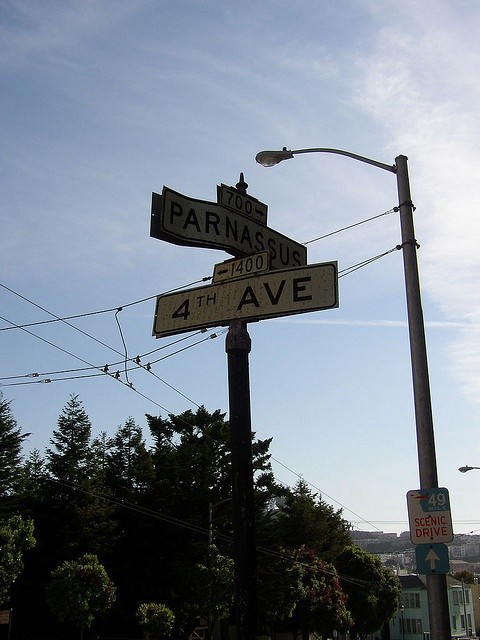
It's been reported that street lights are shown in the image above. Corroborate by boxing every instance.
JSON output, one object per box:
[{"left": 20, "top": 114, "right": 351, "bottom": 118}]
[
  {"left": 255, "top": 147, "right": 452, "bottom": 640},
  {"left": 400, "top": 605, "right": 404, "bottom": 640}
]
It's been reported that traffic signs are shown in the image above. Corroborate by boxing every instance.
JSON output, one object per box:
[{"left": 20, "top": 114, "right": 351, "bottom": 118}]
[
  {"left": 151, "top": 250, "right": 339, "bottom": 336},
  {"left": 160, "top": 183, "right": 307, "bottom": 269}
]
